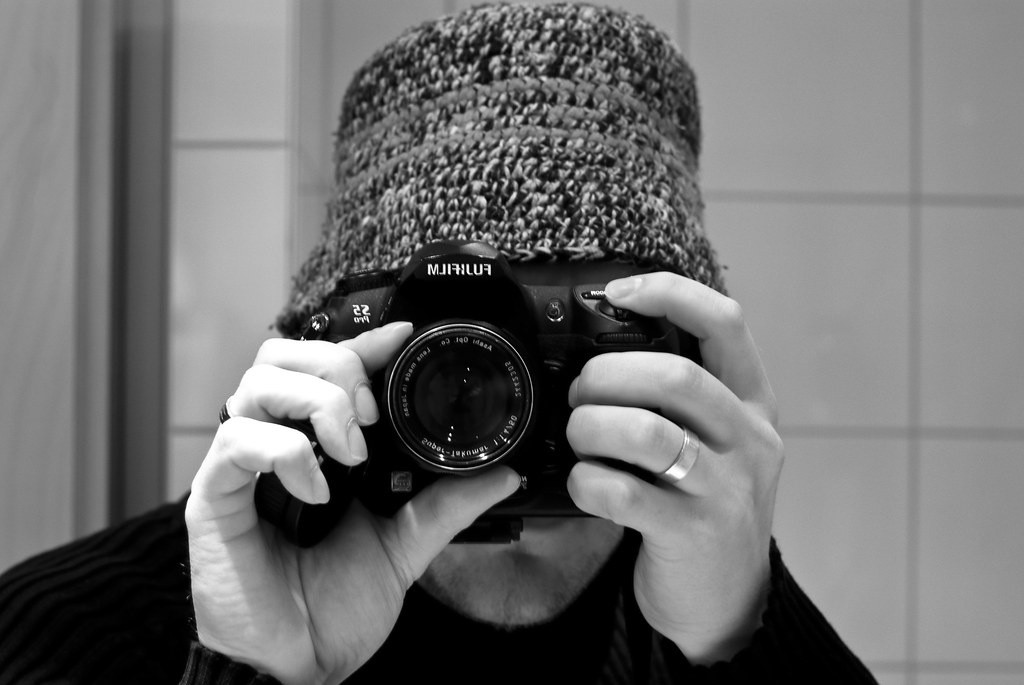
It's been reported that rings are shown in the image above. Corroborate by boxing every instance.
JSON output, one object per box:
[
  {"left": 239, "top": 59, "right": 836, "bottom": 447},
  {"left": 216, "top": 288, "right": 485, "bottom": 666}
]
[
  {"left": 219, "top": 395, "right": 233, "bottom": 424},
  {"left": 654, "top": 424, "right": 701, "bottom": 486}
]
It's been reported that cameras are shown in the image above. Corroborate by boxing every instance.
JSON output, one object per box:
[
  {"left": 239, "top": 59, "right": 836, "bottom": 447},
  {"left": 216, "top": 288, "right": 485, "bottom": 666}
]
[{"left": 314, "top": 239, "right": 679, "bottom": 518}]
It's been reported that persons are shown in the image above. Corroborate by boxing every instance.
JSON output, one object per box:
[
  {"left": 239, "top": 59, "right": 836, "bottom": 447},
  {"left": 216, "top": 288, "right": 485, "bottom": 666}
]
[{"left": 0, "top": 1, "right": 881, "bottom": 685}]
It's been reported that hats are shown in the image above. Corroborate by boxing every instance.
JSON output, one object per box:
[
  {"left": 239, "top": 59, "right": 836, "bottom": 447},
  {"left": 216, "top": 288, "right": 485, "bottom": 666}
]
[{"left": 275, "top": 3, "right": 726, "bottom": 340}]
